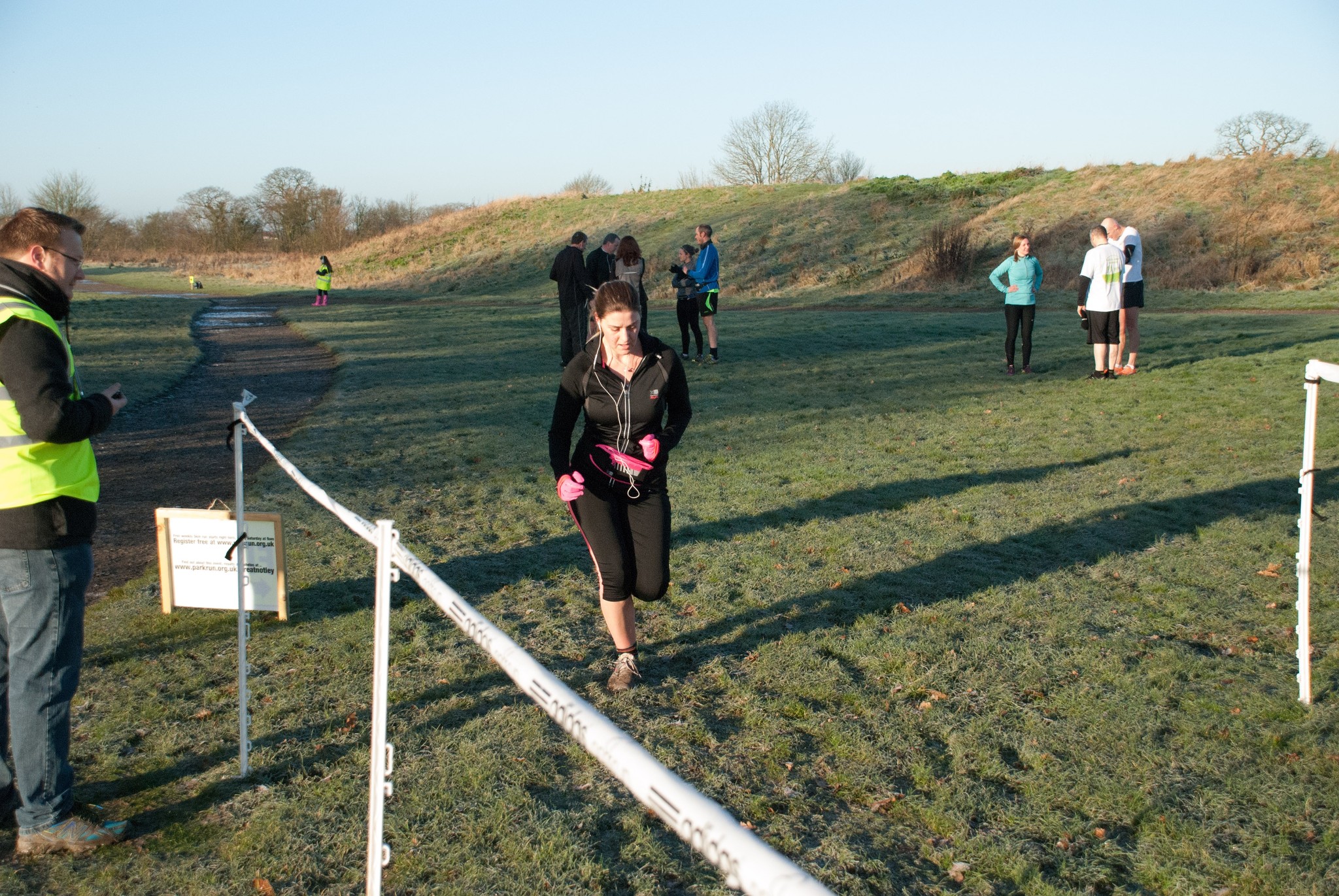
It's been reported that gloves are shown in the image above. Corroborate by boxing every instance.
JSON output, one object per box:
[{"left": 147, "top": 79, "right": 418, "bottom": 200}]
[
  {"left": 675, "top": 264, "right": 686, "bottom": 278},
  {"left": 639, "top": 434, "right": 661, "bottom": 462},
  {"left": 557, "top": 471, "right": 584, "bottom": 501},
  {"left": 668, "top": 266, "right": 677, "bottom": 273}
]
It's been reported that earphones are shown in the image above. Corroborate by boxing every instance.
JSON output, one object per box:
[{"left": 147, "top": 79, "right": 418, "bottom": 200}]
[{"left": 598, "top": 321, "right": 601, "bottom": 331}]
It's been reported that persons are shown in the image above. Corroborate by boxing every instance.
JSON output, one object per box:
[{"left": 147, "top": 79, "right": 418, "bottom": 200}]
[
  {"left": 0, "top": 205, "right": 133, "bottom": 856},
  {"left": 668, "top": 223, "right": 720, "bottom": 367},
  {"left": 670, "top": 245, "right": 704, "bottom": 363},
  {"left": 548, "top": 280, "right": 693, "bottom": 692},
  {"left": 586, "top": 233, "right": 620, "bottom": 294},
  {"left": 608, "top": 235, "right": 649, "bottom": 335},
  {"left": 549, "top": 231, "right": 597, "bottom": 371},
  {"left": 1076, "top": 226, "right": 1126, "bottom": 382},
  {"left": 989, "top": 234, "right": 1043, "bottom": 377},
  {"left": 311, "top": 255, "right": 334, "bottom": 307},
  {"left": 1100, "top": 217, "right": 1144, "bottom": 377}
]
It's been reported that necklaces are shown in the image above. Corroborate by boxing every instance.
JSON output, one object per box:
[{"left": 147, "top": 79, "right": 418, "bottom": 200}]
[{"left": 606, "top": 342, "right": 630, "bottom": 372}]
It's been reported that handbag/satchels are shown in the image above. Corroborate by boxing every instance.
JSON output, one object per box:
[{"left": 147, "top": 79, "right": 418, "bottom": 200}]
[{"left": 587, "top": 444, "right": 654, "bottom": 496}]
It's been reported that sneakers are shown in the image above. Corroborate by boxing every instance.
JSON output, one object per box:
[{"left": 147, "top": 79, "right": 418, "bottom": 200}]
[
  {"left": 1007, "top": 365, "right": 1014, "bottom": 376},
  {"left": 699, "top": 353, "right": 710, "bottom": 362},
  {"left": 692, "top": 354, "right": 704, "bottom": 363},
  {"left": 17, "top": 801, "right": 129, "bottom": 855},
  {"left": 1085, "top": 372, "right": 1105, "bottom": 380},
  {"left": 1021, "top": 365, "right": 1032, "bottom": 373},
  {"left": 0, "top": 782, "right": 27, "bottom": 826},
  {"left": 1104, "top": 367, "right": 1123, "bottom": 376},
  {"left": 698, "top": 354, "right": 720, "bottom": 366},
  {"left": 1118, "top": 366, "right": 1136, "bottom": 375},
  {"left": 680, "top": 353, "right": 689, "bottom": 360},
  {"left": 607, "top": 653, "right": 639, "bottom": 693},
  {"left": 1104, "top": 369, "right": 1117, "bottom": 379}
]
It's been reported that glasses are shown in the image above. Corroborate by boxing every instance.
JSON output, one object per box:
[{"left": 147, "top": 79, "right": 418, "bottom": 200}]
[{"left": 41, "top": 246, "right": 85, "bottom": 270}]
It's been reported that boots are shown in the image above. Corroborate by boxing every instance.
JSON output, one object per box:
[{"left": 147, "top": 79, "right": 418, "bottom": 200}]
[
  {"left": 312, "top": 295, "right": 321, "bottom": 306},
  {"left": 320, "top": 295, "right": 329, "bottom": 306}
]
